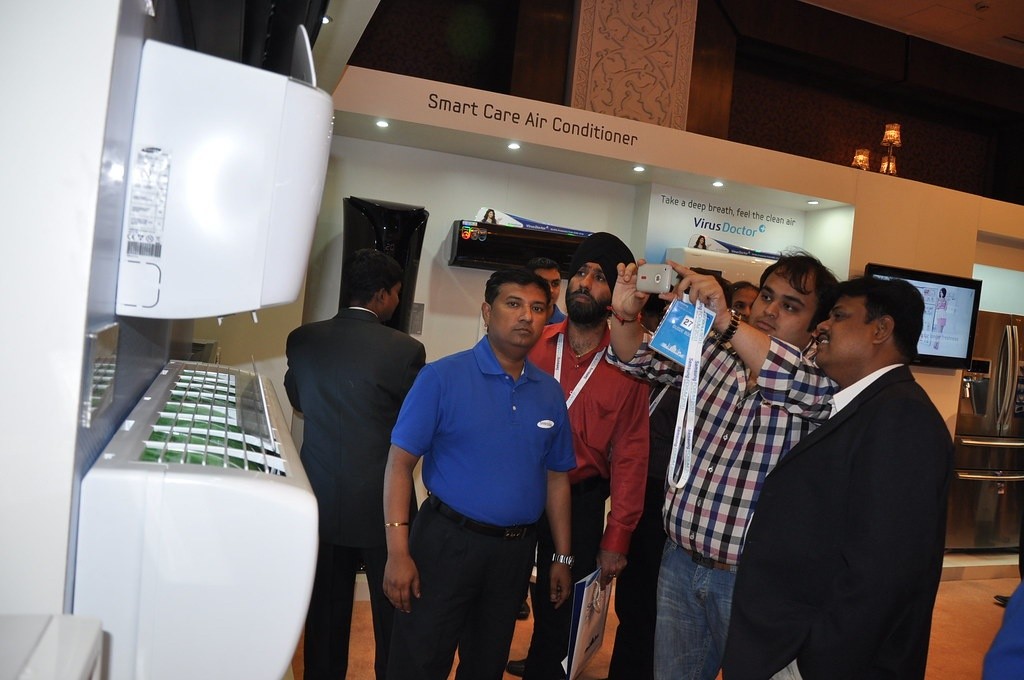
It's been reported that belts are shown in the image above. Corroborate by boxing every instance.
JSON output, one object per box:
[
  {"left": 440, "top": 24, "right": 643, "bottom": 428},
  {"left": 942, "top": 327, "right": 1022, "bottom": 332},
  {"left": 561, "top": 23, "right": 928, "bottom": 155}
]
[
  {"left": 430, "top": 495, "right": 537, "bottom": 540},
  {"left": 668, "top": 532, "right": 739, "bottom": 571}
]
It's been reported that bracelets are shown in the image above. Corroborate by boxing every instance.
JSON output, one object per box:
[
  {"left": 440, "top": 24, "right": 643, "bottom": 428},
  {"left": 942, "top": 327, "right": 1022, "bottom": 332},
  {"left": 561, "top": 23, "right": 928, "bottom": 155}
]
[
  {"left": 607, "top": 306, "right": 641, "bottom": 325},
  {"left": 384, "top": 522, "right": 410, "bottom": 527}
]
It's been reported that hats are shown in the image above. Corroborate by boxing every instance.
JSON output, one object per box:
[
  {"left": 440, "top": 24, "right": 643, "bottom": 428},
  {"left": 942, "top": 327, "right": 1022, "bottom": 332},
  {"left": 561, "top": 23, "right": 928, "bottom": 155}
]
[{"left": 574, "top": 231, "right": 636, "bottom": 296}]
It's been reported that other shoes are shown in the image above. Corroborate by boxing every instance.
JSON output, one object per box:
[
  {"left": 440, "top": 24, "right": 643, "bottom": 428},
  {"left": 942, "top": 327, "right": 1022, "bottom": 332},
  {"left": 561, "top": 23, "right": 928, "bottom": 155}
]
[
  {"left": 518, "top": 601, "right": 530, "bottom": 619},
  {"left": 994, "top": 596, "right": 1012, "bottom": 605},
  {"left": 506, "top": 659, "right": 527, "bottom": 678}
]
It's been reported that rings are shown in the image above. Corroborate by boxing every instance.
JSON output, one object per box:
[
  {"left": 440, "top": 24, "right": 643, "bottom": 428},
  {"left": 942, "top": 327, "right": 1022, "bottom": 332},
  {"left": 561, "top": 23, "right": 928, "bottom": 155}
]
[{"left": 609, "top": 575, "right": 614, "bottom": 578}]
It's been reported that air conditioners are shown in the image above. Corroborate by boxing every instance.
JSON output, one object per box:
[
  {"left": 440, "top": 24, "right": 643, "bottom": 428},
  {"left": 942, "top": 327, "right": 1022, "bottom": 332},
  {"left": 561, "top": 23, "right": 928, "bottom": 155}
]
[
  {"left": 666, "top": 248, "right": 777, "bottom": 290},
  {"left": 448, "top": 220, "right": 588, "bottom": 280},
  {"left": 70, "top": 359, "right": 319, "bottom": 680},
  {"left": 116, "top": 23, "right": 336, "bottom": 323}
]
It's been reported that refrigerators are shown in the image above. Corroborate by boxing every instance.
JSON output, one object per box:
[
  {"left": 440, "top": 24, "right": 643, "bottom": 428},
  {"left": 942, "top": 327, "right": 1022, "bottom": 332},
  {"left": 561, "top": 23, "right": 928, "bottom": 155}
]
[{"left": 944, "top": 310, "right": 1024, "bottom": 548}]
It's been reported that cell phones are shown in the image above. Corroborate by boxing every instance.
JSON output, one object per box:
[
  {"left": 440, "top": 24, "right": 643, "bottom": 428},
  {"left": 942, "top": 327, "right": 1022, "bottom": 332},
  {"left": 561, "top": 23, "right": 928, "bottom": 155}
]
[{"left": 636, "top": 263, "right": 673, "bottom": 293}]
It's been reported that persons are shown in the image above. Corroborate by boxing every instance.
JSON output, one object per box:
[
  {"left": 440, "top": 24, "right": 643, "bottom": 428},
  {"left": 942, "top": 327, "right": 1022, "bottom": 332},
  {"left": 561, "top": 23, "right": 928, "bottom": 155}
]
[
  {"left": 383, "top": 273, "right": 577, "bottom": 680},
  {"left": 715, "top": 275, "right": 955, "bottom": 680},
  {"left": 482, "top": 209, "right": 496, "bottom": 224},
  {"left": 606, "top": 252, "right": 839, "bottom": 680},
  {"left": 694, "top": 236, "right": 706, "bottom": 249},
  {"left": 932, "top": 288, "right": 948, "bottom": 349},
  {"left": 507, "top": 232, "right": 759, "bottom": 680},
  {"left": 284, "top": 251, "right": 427, "bottom": 680}
]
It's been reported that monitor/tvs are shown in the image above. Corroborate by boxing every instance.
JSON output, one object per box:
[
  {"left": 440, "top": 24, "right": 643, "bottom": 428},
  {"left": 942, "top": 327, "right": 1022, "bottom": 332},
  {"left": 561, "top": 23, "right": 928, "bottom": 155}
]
[{"left": 864, "top": 263, "right": 982, "bottom": 372}]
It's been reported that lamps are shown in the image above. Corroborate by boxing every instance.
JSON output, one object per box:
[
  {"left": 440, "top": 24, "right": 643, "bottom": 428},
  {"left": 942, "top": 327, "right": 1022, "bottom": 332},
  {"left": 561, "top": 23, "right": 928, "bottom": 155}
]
[
  {"left": 881, "top": 124, "right": 901, "bottom": 175},
  {"left": 851, "top": 148, "right": 870, "bottom": 172},
  {"left": 879, "top": 156, "right": 897, "bottom": 175}
]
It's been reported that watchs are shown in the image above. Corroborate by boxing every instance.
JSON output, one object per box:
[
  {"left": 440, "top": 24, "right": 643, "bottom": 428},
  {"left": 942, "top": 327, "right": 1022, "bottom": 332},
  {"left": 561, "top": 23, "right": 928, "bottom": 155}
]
[
  {"left": 714, "top": 308, "right": 741, "bottom": 342},
  {"left": 553, "top": 554, "right": 576, "bottom": 567}
]
